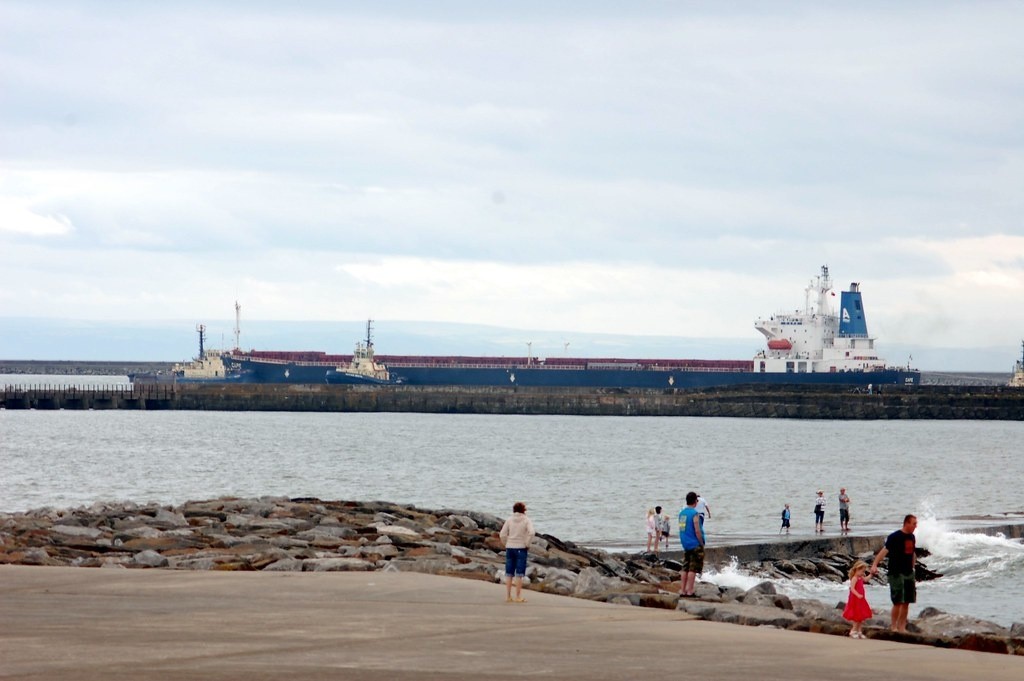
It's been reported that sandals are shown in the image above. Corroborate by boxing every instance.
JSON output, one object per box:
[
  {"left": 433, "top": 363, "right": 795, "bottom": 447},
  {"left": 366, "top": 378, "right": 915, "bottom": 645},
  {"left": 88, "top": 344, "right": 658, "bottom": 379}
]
[{"left": 850, "top": 630, "right": 867, "bottom": 639}]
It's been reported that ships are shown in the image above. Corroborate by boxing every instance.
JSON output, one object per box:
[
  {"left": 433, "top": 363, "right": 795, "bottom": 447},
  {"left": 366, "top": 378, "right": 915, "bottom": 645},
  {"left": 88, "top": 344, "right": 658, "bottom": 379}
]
[{"left": 220, "top": 263, "right": 921, "bottom": 386}]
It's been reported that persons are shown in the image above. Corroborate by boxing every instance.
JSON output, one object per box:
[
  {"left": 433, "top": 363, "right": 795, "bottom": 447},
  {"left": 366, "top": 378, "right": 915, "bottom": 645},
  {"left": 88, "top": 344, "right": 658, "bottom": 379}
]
[
  {"left": 872, "top": 514, "right": 917, "bottom": 633},
  {"left": 695, "top": 495, "right": 711, "bottom": 545},
  {"left": 843, "top": 561, "right": 872, "bottom": 639},
  {"left": 678, "top": 492, "right": 705, "bottom": 598},
  {"left": 814, "top": 490, "right": 824, "bottom": 532},
  {"left": 647, "top": 506, "right": 670, "bottom": 552},
  {"left": 839, "top": 488, "right": 851, "bottom": 530},
  {"left": 780, "top": 503, "right": 791, "bottom": 534},
  {"left": 500, "top": 501, "right": 534, "bottom": 602}
]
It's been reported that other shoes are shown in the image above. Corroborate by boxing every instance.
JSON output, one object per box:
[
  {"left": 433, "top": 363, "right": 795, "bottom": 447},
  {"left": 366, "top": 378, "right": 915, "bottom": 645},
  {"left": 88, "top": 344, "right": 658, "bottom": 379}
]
[
  {"left": 506, "top": 598, "right": 527, "bottom": 602},
  {"left": 680, "top": 592, "right": 701, "bottom": 599}
]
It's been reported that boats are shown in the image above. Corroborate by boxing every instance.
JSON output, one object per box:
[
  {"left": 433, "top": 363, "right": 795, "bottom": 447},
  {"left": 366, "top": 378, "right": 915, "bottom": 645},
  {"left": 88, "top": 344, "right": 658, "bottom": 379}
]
[
  {"left": 323, "top": 320, "right": 407, "bottom": 386},
  {"left": 127, "top": 323, "right": 256, "bottom": 386}
]
